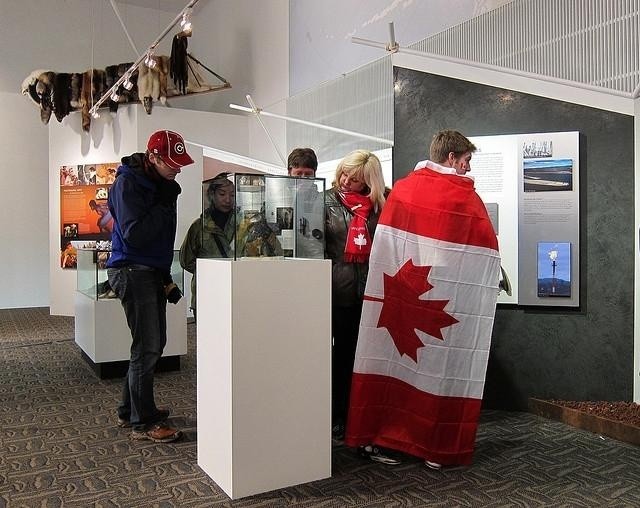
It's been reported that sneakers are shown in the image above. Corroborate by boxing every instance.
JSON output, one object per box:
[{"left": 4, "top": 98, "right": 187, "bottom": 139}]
[
  {"left": 425, "top": 459, "right": 442, "bottom": 470},
  {"left": 332, "top": 438, "right": 345, "bottom": 448},
  {"left": 117, "top": 405, "right": 183, "bottom": 443},
  {"left": 357, "top": 445, "right": 402, "bottom": 465}
]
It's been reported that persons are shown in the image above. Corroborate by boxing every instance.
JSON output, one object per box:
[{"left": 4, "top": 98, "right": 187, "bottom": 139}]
[
  {"left": 179, "top": 180, "right": 235, "bottom": 325},
  {"left": 323, "top": 151, "right": 393, "bottom": 446},
  {"left": 88, "top": 167, "right": 96, "bottom": 185},
  {"left": 344, "top": 130, "right": 499, "bottom": 471},
  {"left": 105, "top": 130, "right": 195, "bottom": 443},
  {"left": 89, "top": 200, "right": 112, "bottom": 232},
  {"left": 63, "top": 243, "right": 76, "bottom": 267},
  {"left": 288, "top": 149, "right": 318, "bottom": 177},
  {"left": 60, "top": 166, "right": 78, "bottom": 186}
]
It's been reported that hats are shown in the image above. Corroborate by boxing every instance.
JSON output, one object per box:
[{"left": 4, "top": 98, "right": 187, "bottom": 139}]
[{"left": 147, "top": 130, "right": 194, "bottom": 170}]
